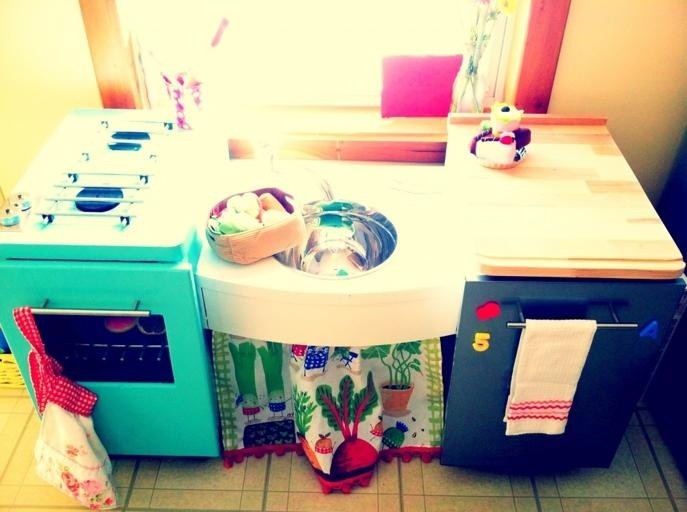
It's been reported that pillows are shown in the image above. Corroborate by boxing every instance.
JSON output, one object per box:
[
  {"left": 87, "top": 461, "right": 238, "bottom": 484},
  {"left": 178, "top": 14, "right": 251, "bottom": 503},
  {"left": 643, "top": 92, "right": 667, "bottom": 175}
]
[{"left": 380, "top": 54, "right": 463, "bottom": 118}]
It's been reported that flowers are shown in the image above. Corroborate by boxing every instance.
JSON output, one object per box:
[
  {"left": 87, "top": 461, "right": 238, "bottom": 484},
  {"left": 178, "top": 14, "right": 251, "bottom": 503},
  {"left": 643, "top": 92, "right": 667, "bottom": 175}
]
[{"left": 454, "top": 0, "right": 524, "bottom": 113}]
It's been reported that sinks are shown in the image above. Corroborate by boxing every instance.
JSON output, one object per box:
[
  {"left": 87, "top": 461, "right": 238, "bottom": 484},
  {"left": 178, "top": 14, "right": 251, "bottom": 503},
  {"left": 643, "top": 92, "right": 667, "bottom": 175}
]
[{"left": 273, "top": 201, "right": 398, "bottom": 280}]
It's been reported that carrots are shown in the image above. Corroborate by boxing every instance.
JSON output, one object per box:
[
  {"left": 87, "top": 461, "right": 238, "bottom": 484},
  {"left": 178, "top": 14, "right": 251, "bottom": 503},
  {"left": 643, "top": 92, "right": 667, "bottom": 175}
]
[{"left": 316, "top": 372, "right": 380, "bottom": 477}]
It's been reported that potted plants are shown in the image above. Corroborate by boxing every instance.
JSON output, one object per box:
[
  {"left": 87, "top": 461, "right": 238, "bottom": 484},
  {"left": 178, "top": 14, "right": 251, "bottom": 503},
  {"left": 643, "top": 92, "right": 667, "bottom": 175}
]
[{"left": 361, "top": 339, "right": 423, "bottom": 416}]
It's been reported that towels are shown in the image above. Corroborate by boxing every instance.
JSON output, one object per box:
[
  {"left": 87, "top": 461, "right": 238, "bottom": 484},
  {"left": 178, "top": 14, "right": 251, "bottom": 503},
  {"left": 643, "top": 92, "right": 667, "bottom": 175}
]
[{"left": 503, "top": 320, "right": 598, "bottom": 436}]
